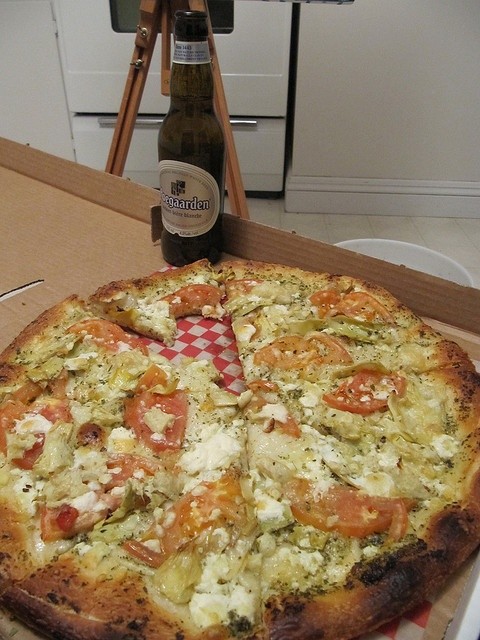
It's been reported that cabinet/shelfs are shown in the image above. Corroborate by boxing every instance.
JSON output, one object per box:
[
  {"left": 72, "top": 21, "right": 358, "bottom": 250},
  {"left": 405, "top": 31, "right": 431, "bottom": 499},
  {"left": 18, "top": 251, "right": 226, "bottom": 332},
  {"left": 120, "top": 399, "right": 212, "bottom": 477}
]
[{"left": 51, "top": 0, "right": 293, "bottom": 193}]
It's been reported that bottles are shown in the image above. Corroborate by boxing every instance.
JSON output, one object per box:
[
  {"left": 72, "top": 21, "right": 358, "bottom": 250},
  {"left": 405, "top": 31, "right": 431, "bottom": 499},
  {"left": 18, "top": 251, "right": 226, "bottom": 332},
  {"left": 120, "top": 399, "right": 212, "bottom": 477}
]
[{"left": 158, "top": 10, "right": 225, "bottom": 267}]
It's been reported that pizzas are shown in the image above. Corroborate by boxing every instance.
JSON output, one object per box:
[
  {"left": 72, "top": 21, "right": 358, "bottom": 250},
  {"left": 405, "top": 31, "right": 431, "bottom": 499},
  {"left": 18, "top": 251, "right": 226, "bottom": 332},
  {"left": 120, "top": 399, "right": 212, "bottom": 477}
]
[{"left": 0, "top": 258, "right": 479, "bottom": 638}]
